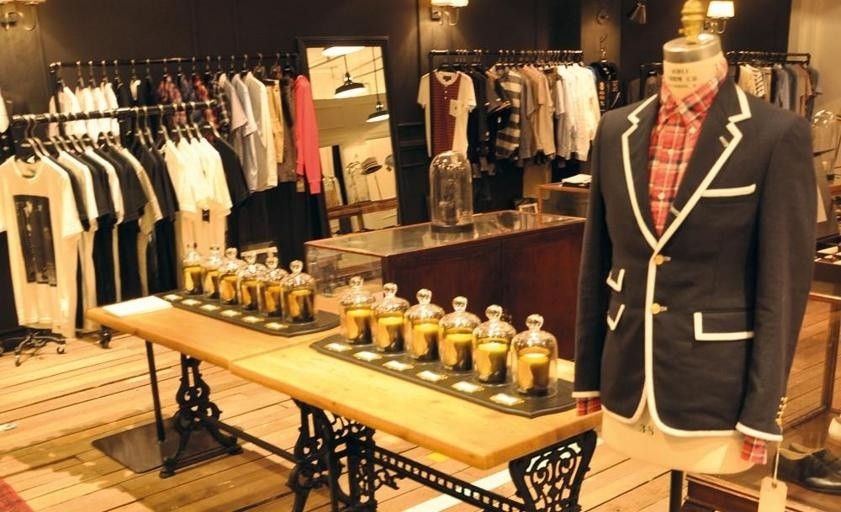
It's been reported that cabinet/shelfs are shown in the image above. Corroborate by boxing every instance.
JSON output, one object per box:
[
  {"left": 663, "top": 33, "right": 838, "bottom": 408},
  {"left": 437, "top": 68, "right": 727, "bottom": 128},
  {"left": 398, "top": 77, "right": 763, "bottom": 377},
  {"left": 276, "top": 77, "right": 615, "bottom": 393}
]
[
  {"left": 300, "top": 210, "right": 586, "bottom": 361},
  {"left": 680, "top": 244, "right": 839, "bottom": 512}
]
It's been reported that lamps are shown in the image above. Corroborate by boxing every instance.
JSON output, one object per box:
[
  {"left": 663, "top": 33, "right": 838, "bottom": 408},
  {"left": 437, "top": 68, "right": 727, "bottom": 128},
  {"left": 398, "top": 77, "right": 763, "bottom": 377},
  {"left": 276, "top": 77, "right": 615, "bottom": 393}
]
[
  {"left": 320, "top": 42, "right": 391, "bottom": 124},
  {"left": 427, "top": 0, "right": 469, "bottom": 28},
  {"left": 703, "top": 0, "right": 735, "bottom": 37}
]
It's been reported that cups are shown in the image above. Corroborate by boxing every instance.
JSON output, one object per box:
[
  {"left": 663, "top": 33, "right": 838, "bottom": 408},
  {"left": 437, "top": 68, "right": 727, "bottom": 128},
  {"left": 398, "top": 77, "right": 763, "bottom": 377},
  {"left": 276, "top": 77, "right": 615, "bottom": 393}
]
[
  {"left": 182, "top": 265, "right": 315, "bottom": 322},
  {"left": 344, "top": 309, "right": 551, "bottom": 398}
]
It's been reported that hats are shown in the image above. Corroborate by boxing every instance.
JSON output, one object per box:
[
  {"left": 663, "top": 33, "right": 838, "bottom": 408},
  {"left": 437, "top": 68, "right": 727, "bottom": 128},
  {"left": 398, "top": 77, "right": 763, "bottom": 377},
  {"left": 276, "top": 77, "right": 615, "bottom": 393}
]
[{"left": 355, "top": 158, "right": 382, "bottom": 176}]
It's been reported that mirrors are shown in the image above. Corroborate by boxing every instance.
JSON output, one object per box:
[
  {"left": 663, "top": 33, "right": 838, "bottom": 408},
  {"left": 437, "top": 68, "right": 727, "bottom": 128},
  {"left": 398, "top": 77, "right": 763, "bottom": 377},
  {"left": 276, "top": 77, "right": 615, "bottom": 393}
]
[{"left": 294, "top": 34, "right": 408, "bottom": 239}]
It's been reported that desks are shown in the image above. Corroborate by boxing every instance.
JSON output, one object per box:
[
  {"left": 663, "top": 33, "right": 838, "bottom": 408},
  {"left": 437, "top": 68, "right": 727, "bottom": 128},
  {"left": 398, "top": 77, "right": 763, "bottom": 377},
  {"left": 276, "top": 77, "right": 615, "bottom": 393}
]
[
  {"left": 83, "top": 286, "right": 340, "bottom": 512},
  {"left": 228, "top": 333, "right": 609, "bottom": 512}
]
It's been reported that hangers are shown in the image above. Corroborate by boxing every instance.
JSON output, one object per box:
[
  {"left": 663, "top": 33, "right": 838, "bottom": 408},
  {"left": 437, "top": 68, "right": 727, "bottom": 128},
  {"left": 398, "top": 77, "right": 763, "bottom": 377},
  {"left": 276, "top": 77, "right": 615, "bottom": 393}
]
[
  {"left": 724, "top": 49, "right": 815, "bottom": 70},
  {"left": 431, "top": 44, "right": 586, "bottom": 75},
  {"left": 0, "top": 54, "right": 296, "bottom": 161}
]
